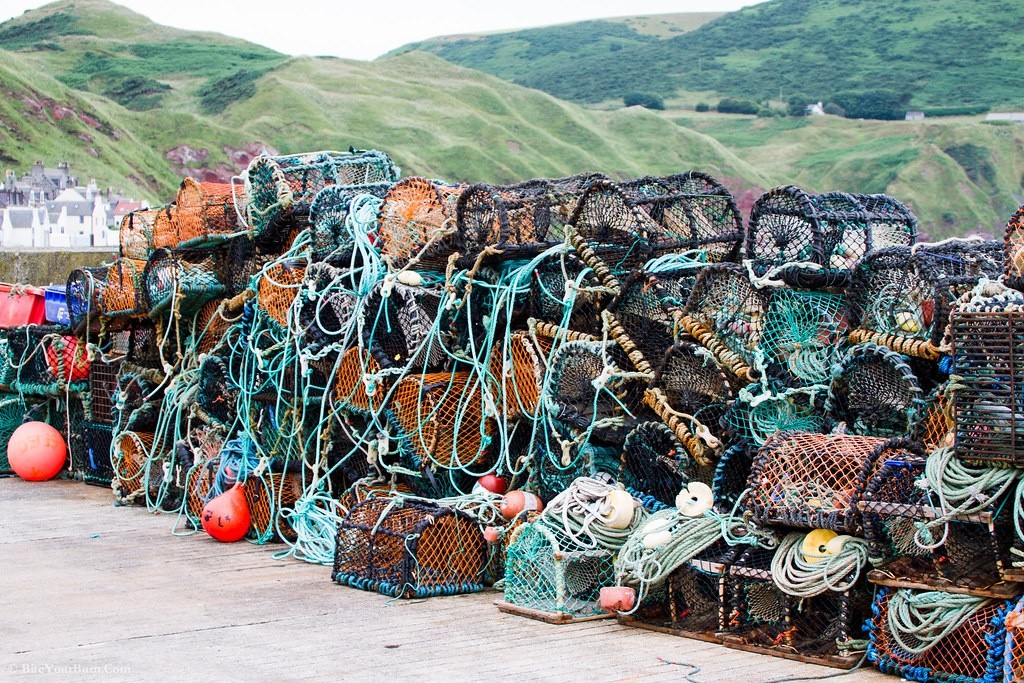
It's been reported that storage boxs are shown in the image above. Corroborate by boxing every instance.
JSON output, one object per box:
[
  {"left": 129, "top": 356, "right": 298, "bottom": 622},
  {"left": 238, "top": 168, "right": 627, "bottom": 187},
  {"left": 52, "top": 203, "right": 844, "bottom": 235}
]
[{"left": 0, "top": 282, "right": 94, "bottom": 330}]
